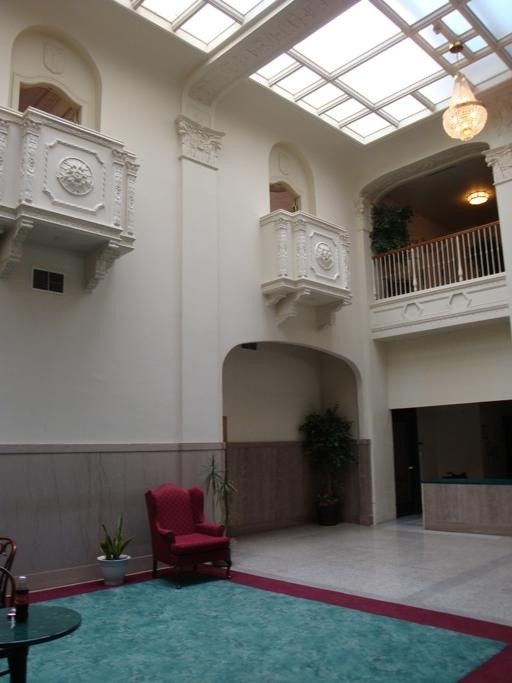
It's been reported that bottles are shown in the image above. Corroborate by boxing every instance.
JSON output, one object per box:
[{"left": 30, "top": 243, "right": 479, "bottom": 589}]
[{"left": 15, "top": 575, "right": 30, "bottom": 622}]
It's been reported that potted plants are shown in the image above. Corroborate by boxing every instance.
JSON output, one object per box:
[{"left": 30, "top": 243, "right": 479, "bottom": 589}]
[
  {"left": 367, "top": 202, "right": 415, "bottom": 296},
  {"left": 95, "top": 512, "right": 133, "bottom": 586},
  {"left": 299, "top": 400, "right": 358, "bottom": 527}
]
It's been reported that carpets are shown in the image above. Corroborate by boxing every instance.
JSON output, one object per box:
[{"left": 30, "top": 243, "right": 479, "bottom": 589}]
[{"left": 1, "top": 563, "right": 512, "bottom": 683}]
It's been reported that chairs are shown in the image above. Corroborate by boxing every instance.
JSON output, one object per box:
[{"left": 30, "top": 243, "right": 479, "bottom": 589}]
[
  {"left": 144, "top": 481, "right": 232, "bottom": 590},
  {"left": 0, "top": 535, "right": 18, "bottom": 682}
]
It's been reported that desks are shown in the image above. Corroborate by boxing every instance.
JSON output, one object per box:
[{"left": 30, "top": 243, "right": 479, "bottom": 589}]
[{"left": 0, "top": 605, "right": 81, "bottom": 683}]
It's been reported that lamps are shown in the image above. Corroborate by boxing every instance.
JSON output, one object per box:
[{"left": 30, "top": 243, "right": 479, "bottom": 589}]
[
  {"left": 441, "top": 39, "right": 489, "bottom": 142},
  {"left": 465, "top": 190, "right": 490, "bottom": 205}
]
